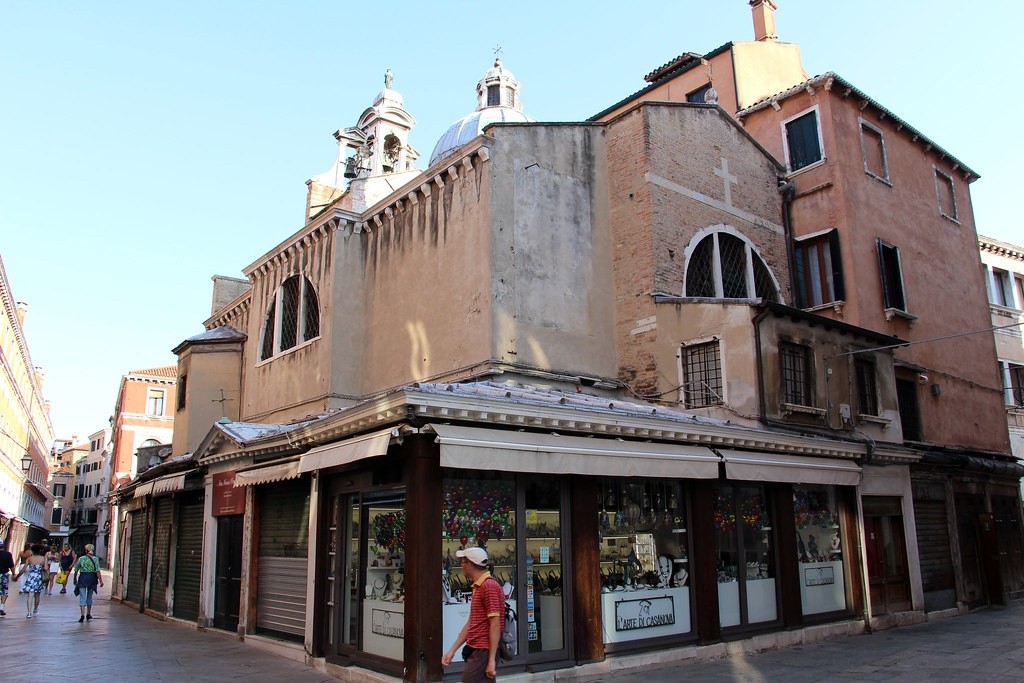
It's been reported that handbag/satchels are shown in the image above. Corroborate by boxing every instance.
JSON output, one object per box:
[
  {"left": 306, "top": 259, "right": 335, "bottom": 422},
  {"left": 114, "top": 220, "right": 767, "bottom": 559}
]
[
  {"left": 56, "top": 568, "right": 67, "bottom": 584},
  {"left": 42, "top": 570, "right": 50, "bottom": 583},
  {"left": 49, "top": 562, "right": 59, "bottom": 575}
]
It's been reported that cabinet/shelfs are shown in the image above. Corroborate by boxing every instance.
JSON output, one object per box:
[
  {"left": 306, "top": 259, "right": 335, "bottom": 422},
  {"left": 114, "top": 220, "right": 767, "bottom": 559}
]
[
  {"left": 349, "top": 505, "right": 630, "bottom": 645},
  {"left": 672, "top": 529, "right": 688, "bottom": 563}
]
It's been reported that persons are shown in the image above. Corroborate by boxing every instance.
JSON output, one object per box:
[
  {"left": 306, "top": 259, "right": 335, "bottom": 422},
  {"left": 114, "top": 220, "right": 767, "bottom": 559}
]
[
  {"left": 13, "top": 545, "right": 47, "bottom": 617},
  {"left": 441, "top": 547, "right": 505, "bottom": 683},
  {"left": 15, "top": 539, "right": 58, "bottom": 593},
  {"left": 74, "top": 544, "right": 104, "bottom": 622},
  {"left": 45, "top": 544, "right": 58, "bottom": 594},
  {"left": 0, "top": 540, "right": 15, "bottom": 617},
  {"left": 59, "top": 543, "right": 76, "bottom": 592}
]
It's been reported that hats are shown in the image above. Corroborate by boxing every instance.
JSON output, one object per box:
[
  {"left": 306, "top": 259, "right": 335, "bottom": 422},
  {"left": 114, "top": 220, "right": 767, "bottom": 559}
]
[
  {"left": 455, "top": 547, "right": 488, "bottom": 567},
  {"left": 0, "top": 540, "right": 6, "bottom": 547}
]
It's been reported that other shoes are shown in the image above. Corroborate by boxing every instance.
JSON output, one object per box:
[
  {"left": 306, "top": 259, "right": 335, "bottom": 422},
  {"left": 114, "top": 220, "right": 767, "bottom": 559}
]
[
  {"left": 60, "top": 588, "right": 66, "bottom": 593},
  {"left": 32, "top": 609, "right": 39, "bottom": 616},
  {"left": 19, "top": 591, "right": 27, "bottom": 595},
  {"left": 0, "top": 604, "right": 7, "bottom": 615},
  {"left": 86, "top": 614, "right": 92, "bottom": 621},
  {"left": 78, "top": 615, "right": 85, "bottom": 622},
  {"left": 45, "top": 590, "right": 52, "bottom": 595},
  {"left": 26, "top": 612, "right": 32, "bottom": 618}
]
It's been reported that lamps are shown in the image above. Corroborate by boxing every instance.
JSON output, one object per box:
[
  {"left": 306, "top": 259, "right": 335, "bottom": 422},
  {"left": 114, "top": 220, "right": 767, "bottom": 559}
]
[
  {"left": 64, "top": 510, "right": 71, "bottom": 527},
  {"left": 0, "top": 427, "right": 33, "bottom": 474}
]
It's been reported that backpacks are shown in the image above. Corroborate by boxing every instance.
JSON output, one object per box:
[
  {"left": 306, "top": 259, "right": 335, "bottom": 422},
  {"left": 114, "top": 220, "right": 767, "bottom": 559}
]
[{"left": 483, "top": 577, "right": 519, "bottom": 662}]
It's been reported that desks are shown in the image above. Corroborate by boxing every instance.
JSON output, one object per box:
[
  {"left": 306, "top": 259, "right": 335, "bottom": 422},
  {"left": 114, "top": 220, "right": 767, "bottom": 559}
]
[
  {"left": 799, "top": 560, "right": 847, "bottom": 615},
  {"left": 717, "top": 578, "right": 777, "bottom": 628},
  {"left": 540, "top": 586, "right": 691, "bottom": 651},
  {"left": 361, "top": 598, "right": 517, "bottom": 663}
]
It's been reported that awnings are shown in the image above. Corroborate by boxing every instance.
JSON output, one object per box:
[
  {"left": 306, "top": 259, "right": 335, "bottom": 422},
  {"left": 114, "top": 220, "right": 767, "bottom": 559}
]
[
  {"left": 297, "top": 425, "right": 403, "bottom": 473},
  {"left": 233, "top": 454, "right": 305, "bottom": 488},
  {"left": 49, "top": 528, "right": 78, "bottom": 536},
  {"left": 424, "top": 424, "right": 862, "bottom": 486},
  {"left": 133, "top": 469, "right": 196, "bottom": 499}
]
